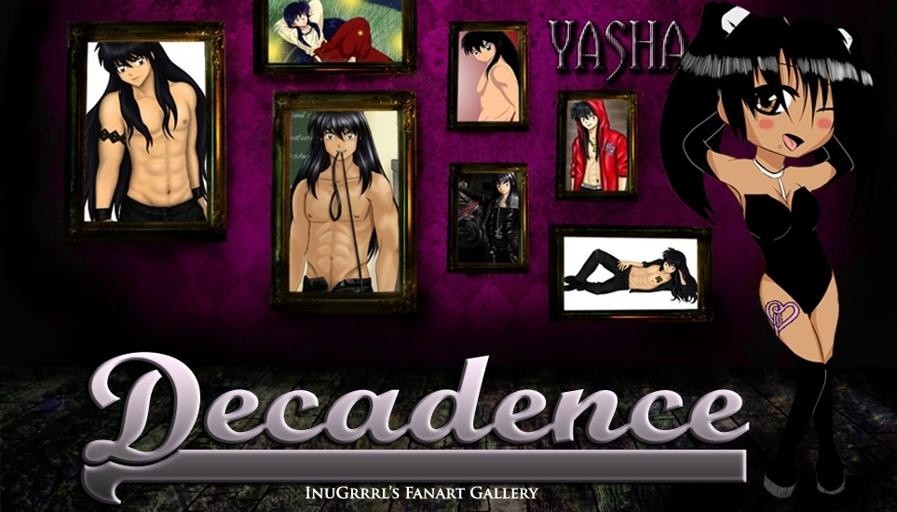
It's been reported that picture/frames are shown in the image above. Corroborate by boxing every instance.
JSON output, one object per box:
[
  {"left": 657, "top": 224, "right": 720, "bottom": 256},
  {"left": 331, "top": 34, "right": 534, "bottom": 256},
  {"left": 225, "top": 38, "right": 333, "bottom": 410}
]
[
  {"left": 447, "top": 20, "right": 531, "bottom": 133},
  {"left": 63, "top": 19, "right": 231, "bottom": 246},
  {"left": 447, "top": 162, "right": 531, "bottom": 276},
  {"left": 548, "top": 222, "right": 716, "bottom": 327},
  {"left": 555, "top": 87, "right": 642, "bottom": 204},
  {"left": 250, "top": 1, "right": 418, "bottom": 317}
]
